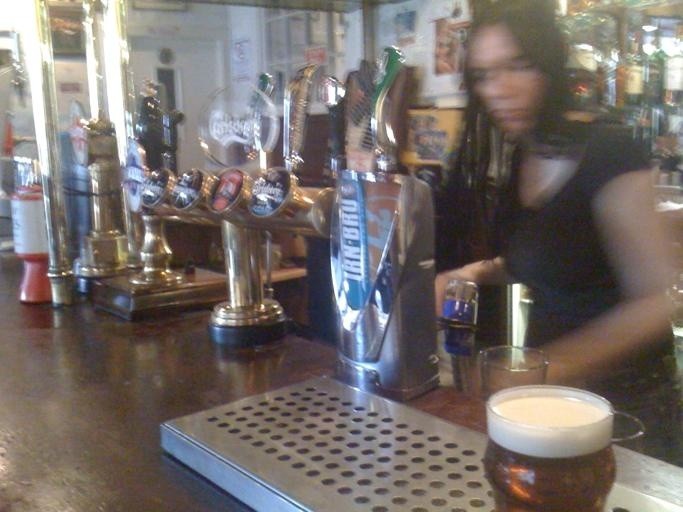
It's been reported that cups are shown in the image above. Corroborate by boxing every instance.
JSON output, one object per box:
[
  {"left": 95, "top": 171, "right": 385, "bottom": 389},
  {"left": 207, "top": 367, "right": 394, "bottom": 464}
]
[
  {"left": 478, "top": 345, "right": 549, "bottom": 406},
  {"left": 7, "top": 185, "right": 53, "bottom": 310},
  {"left": 482, "top": 383, "right": 618, "bottom": 512},
  {"left": 605, "top": 408, "right": 648, "bottom": 453}
]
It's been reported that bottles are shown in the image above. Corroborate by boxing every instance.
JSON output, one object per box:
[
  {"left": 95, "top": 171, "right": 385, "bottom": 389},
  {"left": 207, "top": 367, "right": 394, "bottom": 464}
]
[{"left": 607, "top": 20, "right": 683, "bottom": 187}]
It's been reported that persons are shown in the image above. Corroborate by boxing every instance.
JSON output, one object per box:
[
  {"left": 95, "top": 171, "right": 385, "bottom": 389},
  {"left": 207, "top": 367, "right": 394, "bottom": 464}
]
[{"left": 433, "top": 1, "right": 683, "bottom": 403}]
